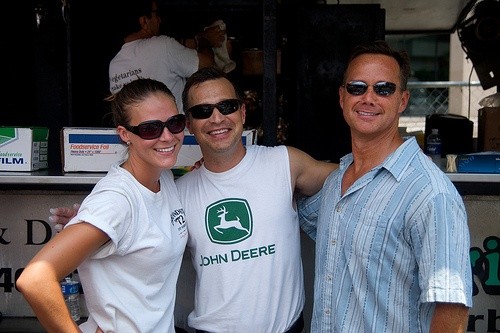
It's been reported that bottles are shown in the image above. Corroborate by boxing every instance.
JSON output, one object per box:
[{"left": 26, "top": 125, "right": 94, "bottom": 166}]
[
  {"left": 427, "top": 129, "right": 441, "bottom": 170},
  {"left": 60, "top": 268, "right": 80, "bottom": 322}
]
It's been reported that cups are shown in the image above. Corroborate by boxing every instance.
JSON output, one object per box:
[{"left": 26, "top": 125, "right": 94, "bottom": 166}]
[{"left": 446, "top": 157, "right": 458, "bottom": 173}]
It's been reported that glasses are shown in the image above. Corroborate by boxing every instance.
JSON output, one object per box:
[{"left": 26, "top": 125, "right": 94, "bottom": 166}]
[
  {"left": 186, "top": 99, "right": 244, "bottom": 119},
  {"left": 341, "top": 80, "right": 405, "bottom": 96},
  {"left": 122, "top": 113, "right": 186, "bottom": 140}
]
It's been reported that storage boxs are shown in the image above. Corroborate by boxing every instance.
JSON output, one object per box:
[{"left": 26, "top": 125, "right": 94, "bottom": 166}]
[
  {"left": 398, "top": 107, "right": 500, "bottom": 173},
  {"left": 0, "top": 126, "right": 49, "bottom": 172},
  {"left": 59, "top": 127, "right": 259, "bottom": 177}
]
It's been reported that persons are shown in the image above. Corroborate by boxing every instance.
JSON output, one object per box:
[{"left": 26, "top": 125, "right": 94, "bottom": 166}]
[
  {"left": 48, "top": 67, "right": 340, "bottom": 333},
  {"left": 15, "top": 78, "right": 188, "bottom": 333},
  {"left": 109, "top": 0, "right": 232, "bottom": 114},
  {"left": 185, "top": 44, "right": 473, "bottom": 333}
]
